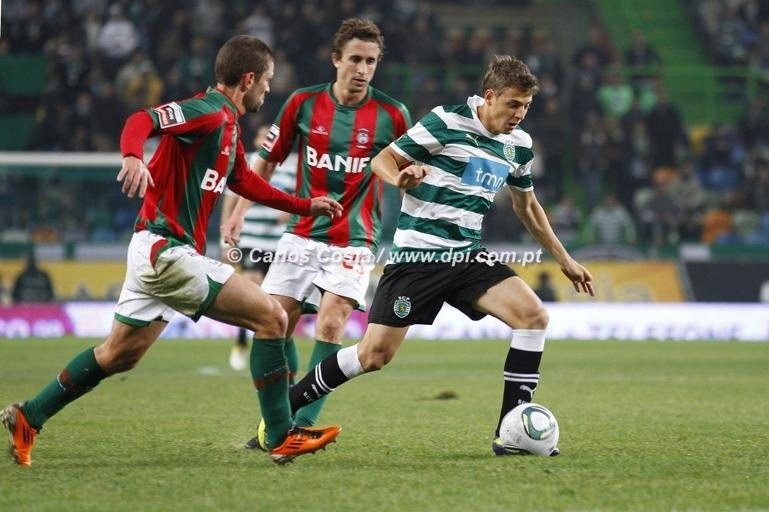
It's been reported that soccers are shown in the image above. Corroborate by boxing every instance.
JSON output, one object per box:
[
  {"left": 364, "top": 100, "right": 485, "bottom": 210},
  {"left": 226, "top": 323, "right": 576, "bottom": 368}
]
[{"left": 500, "top": 403, "right": 559, "bottom": 456}]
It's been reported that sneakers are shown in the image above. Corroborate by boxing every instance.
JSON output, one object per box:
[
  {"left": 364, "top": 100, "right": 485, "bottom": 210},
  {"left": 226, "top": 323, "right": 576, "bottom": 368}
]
[
  {"left": 266, "top": 424, "right": 342, "bottom": 468},
  {"left": 1, "top": 402, "right": 37, "bottom": 468},
  {"left": 492, "top": 437, "right": 562, "bottom": 458}
]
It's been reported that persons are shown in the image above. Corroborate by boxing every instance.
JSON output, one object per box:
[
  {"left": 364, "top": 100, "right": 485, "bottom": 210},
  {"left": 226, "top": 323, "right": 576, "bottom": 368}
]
[
  {"left": 8, "top": 251, "right": 57, "bottom": 302},
  {"left": 1, "top": 1, "right": 767, "bottom": 262},
  {"left": 0, "top": 34, "right": 344, "bottom": 470},
  {"left": 531, "top": 271, "right": 557, "bottom": 303},
  {"left": 218, "top": 16, "right": 418, "bottom": 453},
  {"left": 219, "top": 117, "right": 300, "bottom": 373},
  {"left": 254, "top": 55, "right": 596, "bottom": 455}
]
